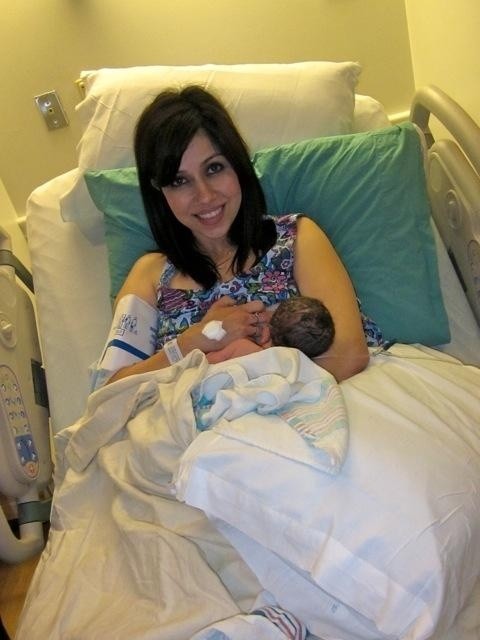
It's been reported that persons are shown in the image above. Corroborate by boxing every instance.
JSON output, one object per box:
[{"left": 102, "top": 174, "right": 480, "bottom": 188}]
[
  {"left": 90, "top": 85, "right": 368, "bottom": 388},
  {"left": 202, "top": 294, "right": 334, "bottom": 363}
]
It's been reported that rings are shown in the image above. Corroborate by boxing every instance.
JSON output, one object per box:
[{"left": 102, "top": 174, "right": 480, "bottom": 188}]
[{"left": 254, "top": 311, "right": 262, "bottom": 325}]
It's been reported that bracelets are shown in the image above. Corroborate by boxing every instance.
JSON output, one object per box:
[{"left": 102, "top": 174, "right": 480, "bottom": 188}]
[{"left": 163, "top": 337, "right": 184, "bottom": 366}]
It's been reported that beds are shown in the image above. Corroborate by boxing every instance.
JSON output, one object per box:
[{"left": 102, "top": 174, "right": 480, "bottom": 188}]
[{"left": 17, "top": 82, "right": 480, "bottom": 639}]
